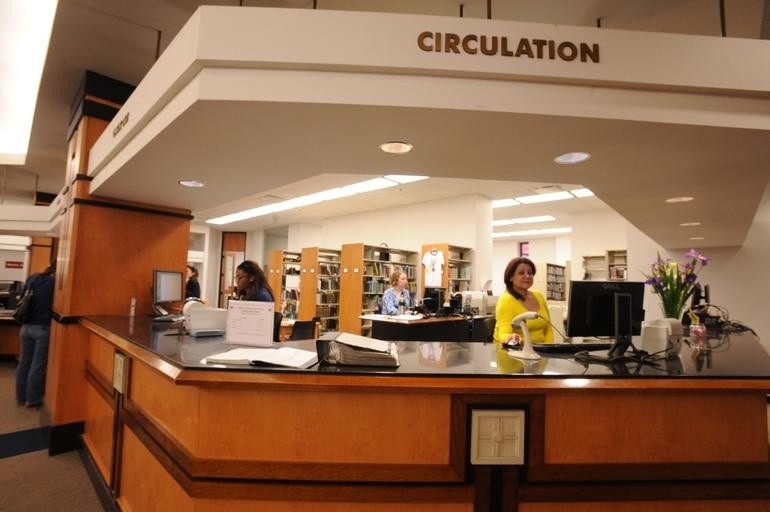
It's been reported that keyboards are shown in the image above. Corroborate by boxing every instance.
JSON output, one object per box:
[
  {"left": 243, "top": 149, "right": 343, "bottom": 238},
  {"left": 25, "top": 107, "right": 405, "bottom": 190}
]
[
  {"left": 533, "top": 343, "right": 612, "bottom": 351},
  {"left": 152, "top": 313, "right": 185, "bottom": 322}
]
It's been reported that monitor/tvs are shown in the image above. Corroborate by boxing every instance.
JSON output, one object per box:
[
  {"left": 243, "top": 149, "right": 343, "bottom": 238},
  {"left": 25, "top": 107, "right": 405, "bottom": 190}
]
[
  {"left": 566, "top": 279, "right": 646, "bottom": 363},
  {"left": 423, "top": 287, "right": 446, "bottom": 313},
  {"left": 152, "top": 270, "right": 184, "bottom": 315}
]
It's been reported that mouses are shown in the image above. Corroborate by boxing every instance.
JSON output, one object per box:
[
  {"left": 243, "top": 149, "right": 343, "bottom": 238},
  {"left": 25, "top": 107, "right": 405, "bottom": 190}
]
[{"left": 507, "top": 338, "right": 519, "bottom": 346}]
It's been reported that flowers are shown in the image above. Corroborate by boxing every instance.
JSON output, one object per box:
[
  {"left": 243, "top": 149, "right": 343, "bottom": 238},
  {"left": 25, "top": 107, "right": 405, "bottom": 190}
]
[{"left": 637, "top": 246, "right": 712, "bottom": 319}]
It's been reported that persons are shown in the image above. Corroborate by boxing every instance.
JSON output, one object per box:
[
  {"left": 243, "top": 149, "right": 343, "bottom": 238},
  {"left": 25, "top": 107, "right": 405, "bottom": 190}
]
[
  {"left": 236, "top": 260, "right": 274, "bottom": 304},
  {"left": 14, "top": 259, "right": 57, "bottom": 408},
  {"left": 380, "top": 271, "right": 416, "bottom": 315},
  {"left": 493, "top": 256, "right": 555, "bottom": 346},
  {"left": 184, "top": 265, "right": 200, "bottom": 303}
]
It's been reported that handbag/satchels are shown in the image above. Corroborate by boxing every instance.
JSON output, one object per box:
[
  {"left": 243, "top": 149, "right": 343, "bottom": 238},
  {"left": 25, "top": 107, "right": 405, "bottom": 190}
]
[{"left": 11, "top": 291, "right": 34, "bottom": 323}]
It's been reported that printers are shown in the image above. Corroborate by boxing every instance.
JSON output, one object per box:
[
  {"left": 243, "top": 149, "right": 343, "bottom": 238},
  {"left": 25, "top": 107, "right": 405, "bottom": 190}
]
[
  {"left": 182, "top": 300, "right": 228, "bottom": 337},
  {"left": 626, "top": 317, "right": 684, "bottom": 360}
]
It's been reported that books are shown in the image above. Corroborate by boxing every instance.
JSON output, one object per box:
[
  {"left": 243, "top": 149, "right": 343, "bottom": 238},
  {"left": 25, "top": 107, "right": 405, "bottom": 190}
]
[{"left": 207, "top": 346, "right": 320, "bottom": 369}]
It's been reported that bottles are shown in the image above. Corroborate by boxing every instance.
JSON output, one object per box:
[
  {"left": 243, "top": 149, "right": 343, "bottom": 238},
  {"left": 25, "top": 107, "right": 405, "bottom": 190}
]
[{"left": 398, "top": 295, "right": 406, "bottom": 316}]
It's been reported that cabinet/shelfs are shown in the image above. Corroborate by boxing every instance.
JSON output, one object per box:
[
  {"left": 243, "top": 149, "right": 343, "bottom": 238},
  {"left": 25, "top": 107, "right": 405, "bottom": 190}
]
[
  {"left": 270, "top": 247, "right": 302, "bottom": 324},
  {"left": 341, "top": 246, "right": 417, "bottom": 336},
  {"left": 421, "top": 245, "right": 472, "bottom": 304},
  {"left": 585, "top": 249, "right": 626, "bottom": 280},
  {"left": 302, "top": 249, "right": 340, "bottom": 331},
  {"left": 546, "top": 265, "right": 568, "bottom": 301}
]
